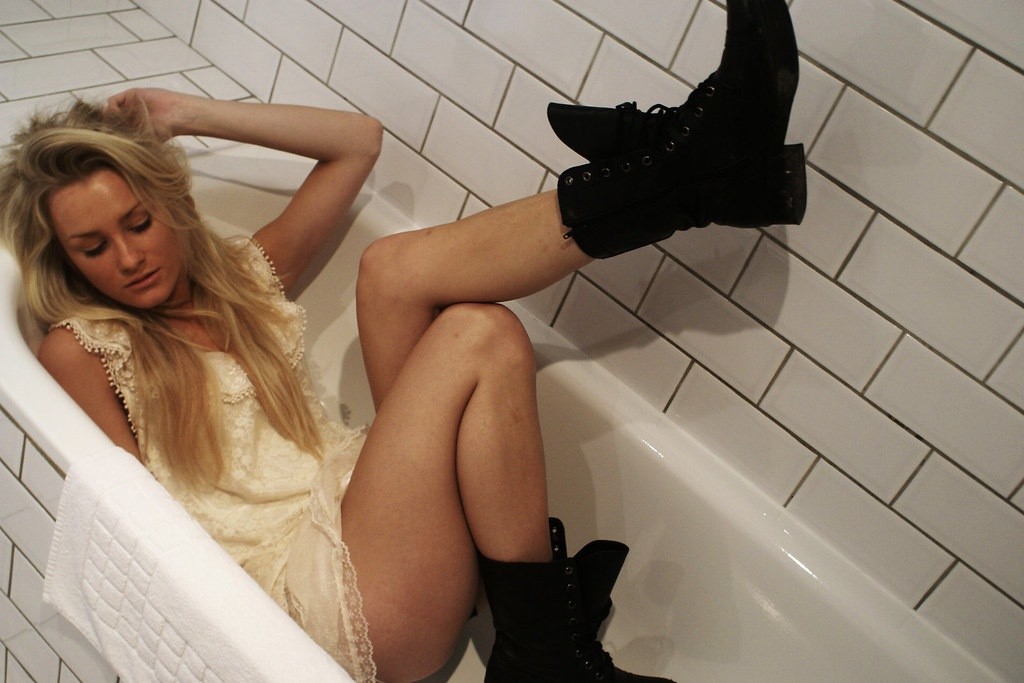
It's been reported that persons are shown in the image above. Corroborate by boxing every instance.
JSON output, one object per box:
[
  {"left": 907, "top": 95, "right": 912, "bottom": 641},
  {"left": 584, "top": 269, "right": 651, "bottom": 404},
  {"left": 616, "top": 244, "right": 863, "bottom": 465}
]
[{"left": 0, "top": 0, "right": 808, "bottom": 683}]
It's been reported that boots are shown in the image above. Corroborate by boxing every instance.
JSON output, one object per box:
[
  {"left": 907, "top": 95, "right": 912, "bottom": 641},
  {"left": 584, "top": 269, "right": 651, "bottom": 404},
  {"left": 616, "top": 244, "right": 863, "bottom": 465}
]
[
  {"left": 471, "top": 516, "right": 677, "bottom": 683},
  {"left": 547, "top": 0, "right": 807, "bottom": 259}
]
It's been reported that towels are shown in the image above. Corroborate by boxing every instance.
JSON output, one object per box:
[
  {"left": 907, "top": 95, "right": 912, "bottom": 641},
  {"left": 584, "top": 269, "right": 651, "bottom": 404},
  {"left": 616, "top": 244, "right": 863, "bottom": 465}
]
[{"left": 41, "top": 446, "right": 355, "bottom": 683}]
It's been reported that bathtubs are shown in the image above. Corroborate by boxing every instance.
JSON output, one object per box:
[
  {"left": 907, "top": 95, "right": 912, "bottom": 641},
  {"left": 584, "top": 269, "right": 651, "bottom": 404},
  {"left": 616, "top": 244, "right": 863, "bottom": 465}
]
[{"left": 0, "top": 133, "right": 1000, "bottom": 681}]
